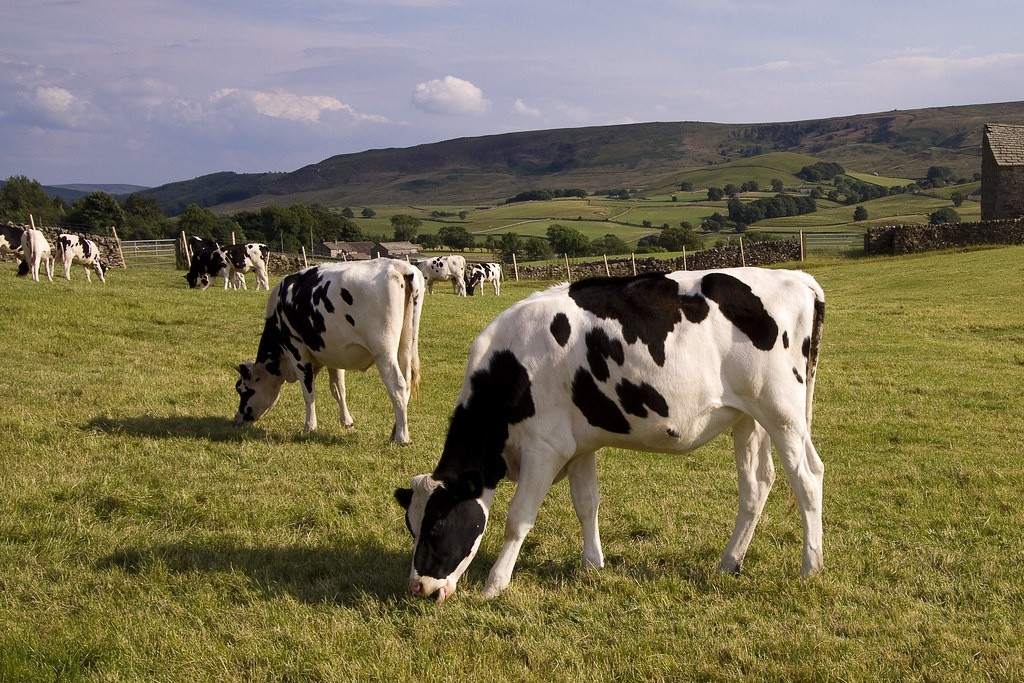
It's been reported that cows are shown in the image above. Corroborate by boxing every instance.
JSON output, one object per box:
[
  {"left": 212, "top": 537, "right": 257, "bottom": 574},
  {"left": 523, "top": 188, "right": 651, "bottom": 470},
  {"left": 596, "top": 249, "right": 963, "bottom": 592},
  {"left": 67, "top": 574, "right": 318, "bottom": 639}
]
[
  {"left": 232, "top": 257, "right": 427, "bottom": 449},
  {"left": 417, "top": 255, "right": 505, "bottom": 297},
  {"left": 182, "top": 235, "right": 271, "bottom": 291},
  {"left": 396, "top": 265, "right": 827, "bottom": 608},
  {"left": 0, "top": 225, "right": 107, "bottom": 285}
]
